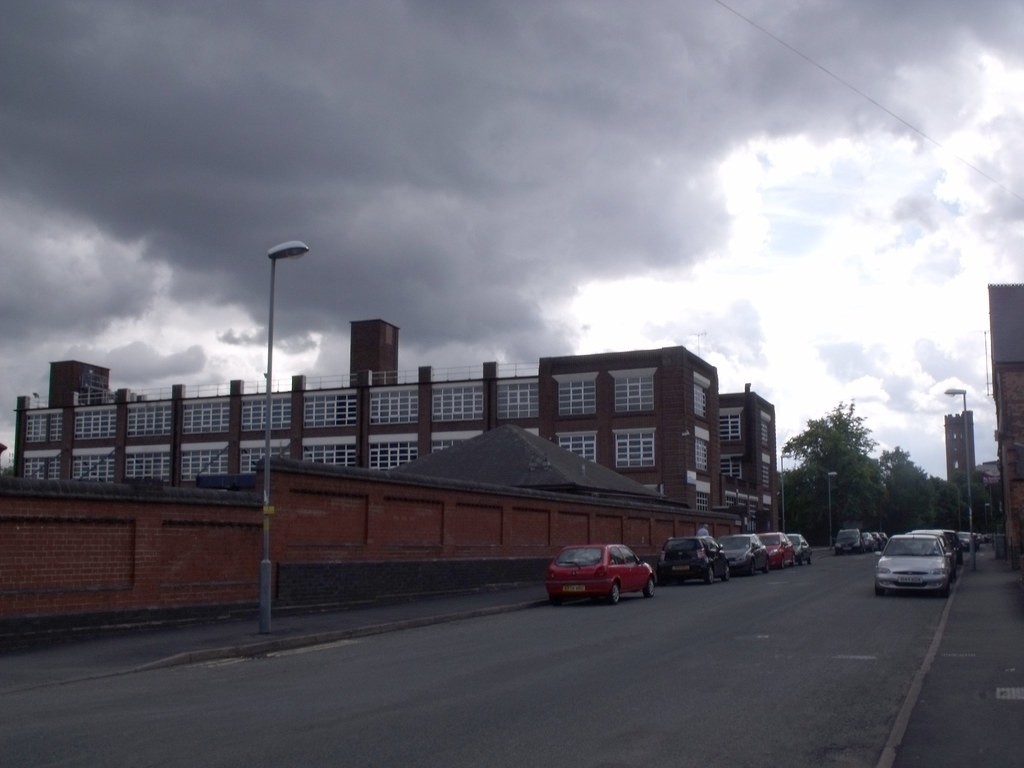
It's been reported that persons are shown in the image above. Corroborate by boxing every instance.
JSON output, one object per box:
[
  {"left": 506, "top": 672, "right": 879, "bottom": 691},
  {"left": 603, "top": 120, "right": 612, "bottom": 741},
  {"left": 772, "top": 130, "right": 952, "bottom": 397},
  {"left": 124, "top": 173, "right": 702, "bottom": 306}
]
[{"left": 697, "top": 524, "right": 709, "bottom": 536}]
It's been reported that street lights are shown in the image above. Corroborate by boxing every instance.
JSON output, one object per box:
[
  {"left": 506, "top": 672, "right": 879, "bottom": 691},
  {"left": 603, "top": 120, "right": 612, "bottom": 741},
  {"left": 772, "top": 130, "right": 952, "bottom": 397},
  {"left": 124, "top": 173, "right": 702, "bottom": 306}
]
[
  {"left": 246, "top": 241, "right": 312, "bottom": 635},
  {"left": 944, "top": 389, "right": 976, "bottom": 571},
  {"left": 781, "top": 454, "right": 793, "bottom": 531},
  {"left": 827, "top": 471, "right": 839, "bottom": 550},
  {"left": 984, "top": 503, "right": 991, "bottom": 534}
]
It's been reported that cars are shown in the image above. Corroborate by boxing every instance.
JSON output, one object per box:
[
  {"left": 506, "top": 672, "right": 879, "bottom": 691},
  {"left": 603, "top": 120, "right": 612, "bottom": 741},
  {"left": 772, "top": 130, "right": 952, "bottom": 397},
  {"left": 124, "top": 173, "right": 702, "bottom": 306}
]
[
  {"left": 861, "top": 531, "right": 888, "bottom": 552},
  {"left": 716, "top": 533, "right": 770, "bottom": 576},
  {"left": 834, "top": 528, "right": 864, "bottom": 555},
  {"left": 874, "top": 534, "right": 953, "bottom": 598},
  {"left": 757, "top": 532, "right": 795, "bottom": 569},
  {"left": 904, "top": 529, "right": 992, "bottom": 578},
  {"left": 656, "top": 535, "right": 731, "bottom": 585},
  {"left": 544, "top": 543, "right": 657, "bottom": 607},
  {"left": 786, "top": 533, "right": 812, "bottom": 567}
]
